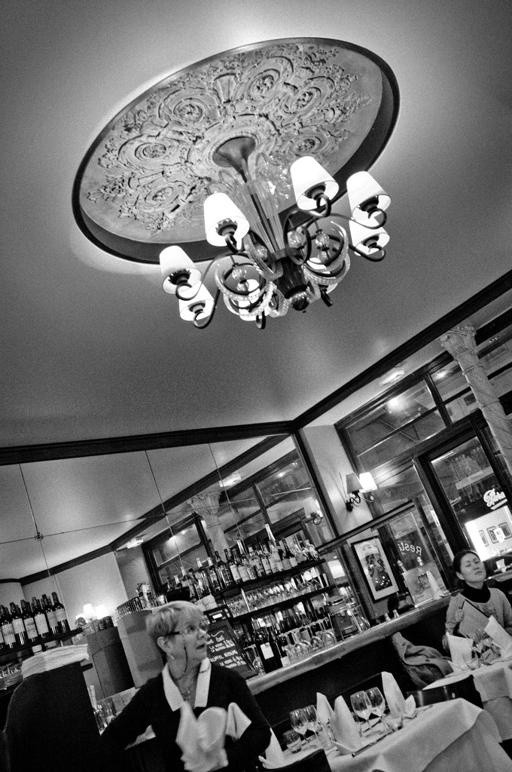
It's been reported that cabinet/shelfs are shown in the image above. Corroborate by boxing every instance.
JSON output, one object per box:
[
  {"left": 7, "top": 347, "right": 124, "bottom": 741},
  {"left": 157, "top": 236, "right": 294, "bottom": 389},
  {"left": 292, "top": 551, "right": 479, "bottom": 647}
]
[{"left": 194, "top": 558, "right": 334, "bottom": 672}]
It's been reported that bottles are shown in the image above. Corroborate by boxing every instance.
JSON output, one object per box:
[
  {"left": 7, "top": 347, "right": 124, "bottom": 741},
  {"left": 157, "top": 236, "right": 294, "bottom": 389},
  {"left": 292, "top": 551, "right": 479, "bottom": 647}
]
[
  {"left": 225, "top": 548, "right": 241, "bottom": 584},
  {"left": 173, "top": 574, "right": 182, "bottom": 589},
  {"left": 196, "top": 557, "right": 212, "bottom": 593},
  {"left": 282, "top": 536, "right": 297, "bottom": 567},
  {"left": 180, "top": 565, "right": 197, "bottom": 600},
  {"left": 253, "top": 540, "right": 271, "bottom": 575},
  {"left": 0, "top": 604, "right": 17, "bottom": 654},
  {"left": 20, "top": 600, "right": 40, "bottom": 644},
  {"left": 276, "top": 591, "right": 360, "bottom": 651},
  {"left": 274, "top": 539, "right": 289, "bottom": 570},
  {"left": 253, "top": 615, "right": 283, "bottom": 673},
  {"left": 166, "top": 576, "right": 173, "bottom": 592},
  {"left": 41, "top": 593, "right": 61, "bottom": 637},
  {"left": 247, "top": 544, "right": 263, "bottom": 577},
  {"left": 269, "top": 538, "right": 282, "bottom": 571},
  {"left": 214, "top": 550, "right": 232, "bottom": 589},
  {"left": 231, "top": 547, "right": 249, "bottom": 582},
  {"left": 206, "top": 555, "right": 222, "bottom": 591},
  {"left": 258, "top": 540, "right": 277, "bottom": 574},
  {"left": 52, "top": 591, "right": 70, "bottom": 633},
  {"left": 10, "top": 602, "right": 28, "bottom": 650},
  {"left": 237, "top": 538, "right": 256, "bottom": 581},
  {"left": 31, "top": 596, "right": 52, "bottom": 641}
]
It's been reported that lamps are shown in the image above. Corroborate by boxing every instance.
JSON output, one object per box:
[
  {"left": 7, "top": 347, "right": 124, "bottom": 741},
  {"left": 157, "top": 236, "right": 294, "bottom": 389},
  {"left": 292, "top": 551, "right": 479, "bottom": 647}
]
[
  {"left": 187, "top": 481, "right": 233, "bottom": 563},
  {"left": 345, "top": 471, "right": 377, "bottom": 512},
  {"left": 83, "top": 602, "right": 95, "bottom": 620},
  {"left": 157, "top": 133, "right": 392, "bottom": 333}
]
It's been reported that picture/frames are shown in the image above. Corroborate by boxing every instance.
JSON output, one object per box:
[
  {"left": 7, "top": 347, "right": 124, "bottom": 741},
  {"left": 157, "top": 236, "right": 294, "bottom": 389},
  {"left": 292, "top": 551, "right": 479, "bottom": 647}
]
[
  {"left": 486, "top": 526, "right": 501, "bottom": 544},
  {"left": 498, "top": 522, "right": 512, "bottom": 539},
  {"left": 478, "top": 529, "right": 490, "bottom": 547},
  {"left": 352, "top": 535, "right": 399, "bottom": 604}
]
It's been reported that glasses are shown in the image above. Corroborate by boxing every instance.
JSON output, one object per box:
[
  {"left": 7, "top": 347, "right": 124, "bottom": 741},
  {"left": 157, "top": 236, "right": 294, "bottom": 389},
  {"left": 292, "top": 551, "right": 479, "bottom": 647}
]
[{"left": 165, "top": 622, "right": 213, "bottom": 637}]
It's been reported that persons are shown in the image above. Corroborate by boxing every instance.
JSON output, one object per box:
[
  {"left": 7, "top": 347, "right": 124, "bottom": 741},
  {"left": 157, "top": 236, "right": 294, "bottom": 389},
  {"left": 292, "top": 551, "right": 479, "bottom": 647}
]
[
  {"left": 443, "top": 549, "right": 512, "bottom": 654},
  {"left": 72, "top": 600, "right": 271, "bottom": 772}
]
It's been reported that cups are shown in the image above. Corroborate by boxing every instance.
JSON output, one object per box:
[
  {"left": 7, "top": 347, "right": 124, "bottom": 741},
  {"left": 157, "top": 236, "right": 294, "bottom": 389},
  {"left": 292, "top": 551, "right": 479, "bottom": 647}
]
[
  {"left": 302, "top": 704, "right": 328, "bottom": 750},
  {"left": 313, "top": 617, "right": 337, "bottom": 650},
  {"left": 465, "top": 629, "right": 497, "bottom": 670},
  {"left": 279, "top": 628, "right": 312, "bottom": 664},
  {"left": 365, "top": 686, "right": 396, "bottom": 734},
  {"left": 349, "top": 690, "right": 385, "bottom": 739},
  {"left": 461, "top": 647, "right": 485, "bottom": 672},
  {"left": 379, "top": 702, "right": 404, "bottom": 733},
  {"left": 282, "top": 729, "right": 303, "bottom": 755},
  {"left": 475, "top": 626, "right": 507, "bottom": 663},
  {"left": 302, "top": 621, "right": 326, "bottom": 653},
  {"left": 288, "top": 707, "right": 317, "bottom": 751}
]
